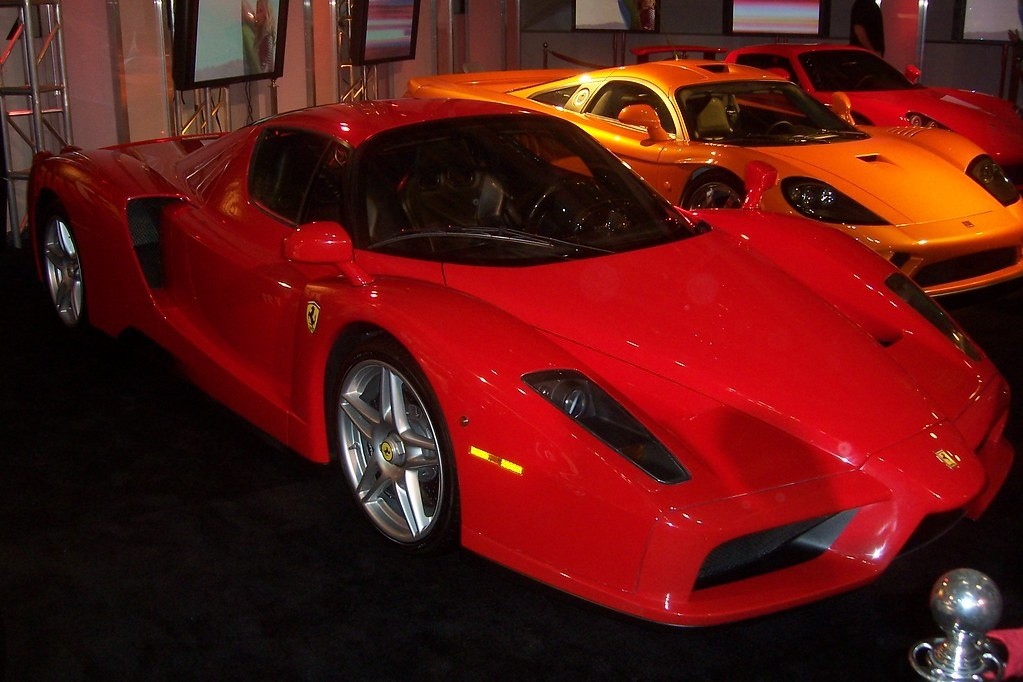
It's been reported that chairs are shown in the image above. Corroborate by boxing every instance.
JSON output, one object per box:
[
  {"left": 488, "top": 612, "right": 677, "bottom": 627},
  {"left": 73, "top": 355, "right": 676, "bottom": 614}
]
[
  {"left": 313, "top": 139, "right": 507, "bottom": 254},
  {"left": 623, "top": 95, "right": 732, "bottom": 136}
]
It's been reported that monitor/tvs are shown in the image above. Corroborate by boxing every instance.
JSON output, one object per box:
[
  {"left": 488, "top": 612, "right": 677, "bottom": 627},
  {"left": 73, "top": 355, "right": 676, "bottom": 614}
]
[
  {"left": 171, "top": 0, "right": 290, "bottom": 91},
  {"left": 350, "top": 0, "right": 421, "bottom": 66},
  {"left": 570, "top": 0, "right": 662, "bottom": 33},
  {"left": 721, "top": 0, "right": 832, "bottom": 40}
]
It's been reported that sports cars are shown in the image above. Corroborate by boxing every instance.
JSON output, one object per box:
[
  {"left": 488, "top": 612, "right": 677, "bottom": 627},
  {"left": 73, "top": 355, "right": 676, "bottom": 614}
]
[
  {"left": 629, "top": 43, "right": 1023, "bottom": 194},
  {"left": 26, "top": 97, "right": 1013, "bottom": 628},
  {"left": 406, "top": 58, "right": 1023, "bottom": 299}
]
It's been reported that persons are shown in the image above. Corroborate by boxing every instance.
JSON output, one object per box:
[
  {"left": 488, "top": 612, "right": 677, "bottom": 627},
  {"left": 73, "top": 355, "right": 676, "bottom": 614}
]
[
  {"left": 849, "top": 0, "right": 886, "bottom": 58},
  {"left": 1009, "top": 30, "right": 1023, "bottom": 46},
  {"left": 242, "top": 0, "right": 275, "bottom": 75}
]
